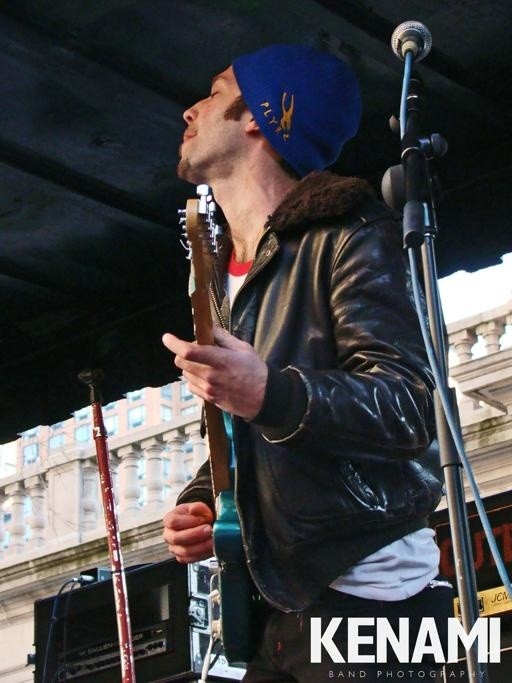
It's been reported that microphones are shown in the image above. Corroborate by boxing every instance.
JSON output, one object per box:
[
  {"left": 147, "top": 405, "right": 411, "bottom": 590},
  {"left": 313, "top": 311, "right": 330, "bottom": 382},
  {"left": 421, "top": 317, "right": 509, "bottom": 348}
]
[{"left": 390, "top": 19, "right": 433, "bottom": 139}]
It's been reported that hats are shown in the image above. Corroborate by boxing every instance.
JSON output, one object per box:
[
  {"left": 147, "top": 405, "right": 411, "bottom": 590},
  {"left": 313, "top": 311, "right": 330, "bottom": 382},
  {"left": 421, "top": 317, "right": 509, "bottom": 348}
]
[{"left": 232, "top": 43, "right": 364, "bottom": 179}]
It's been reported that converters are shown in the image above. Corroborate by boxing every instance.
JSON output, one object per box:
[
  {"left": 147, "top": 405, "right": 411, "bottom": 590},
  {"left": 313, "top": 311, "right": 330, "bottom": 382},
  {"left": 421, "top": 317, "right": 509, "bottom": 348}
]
[{"left": 80, "top": 568, "right": 112, "bottom": 587}]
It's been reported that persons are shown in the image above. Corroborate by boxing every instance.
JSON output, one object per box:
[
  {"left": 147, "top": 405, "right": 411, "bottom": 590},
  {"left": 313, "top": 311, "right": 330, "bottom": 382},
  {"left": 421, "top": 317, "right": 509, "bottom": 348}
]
[{"left": 161, "top": 43, "right": 455, "bottom": 683}]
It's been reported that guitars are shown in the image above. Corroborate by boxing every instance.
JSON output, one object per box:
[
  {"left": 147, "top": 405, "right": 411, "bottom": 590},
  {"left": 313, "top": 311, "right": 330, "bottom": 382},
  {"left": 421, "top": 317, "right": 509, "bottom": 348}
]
[{"left": 176, "top": 184, "right": 255, "bottom": 670}]
